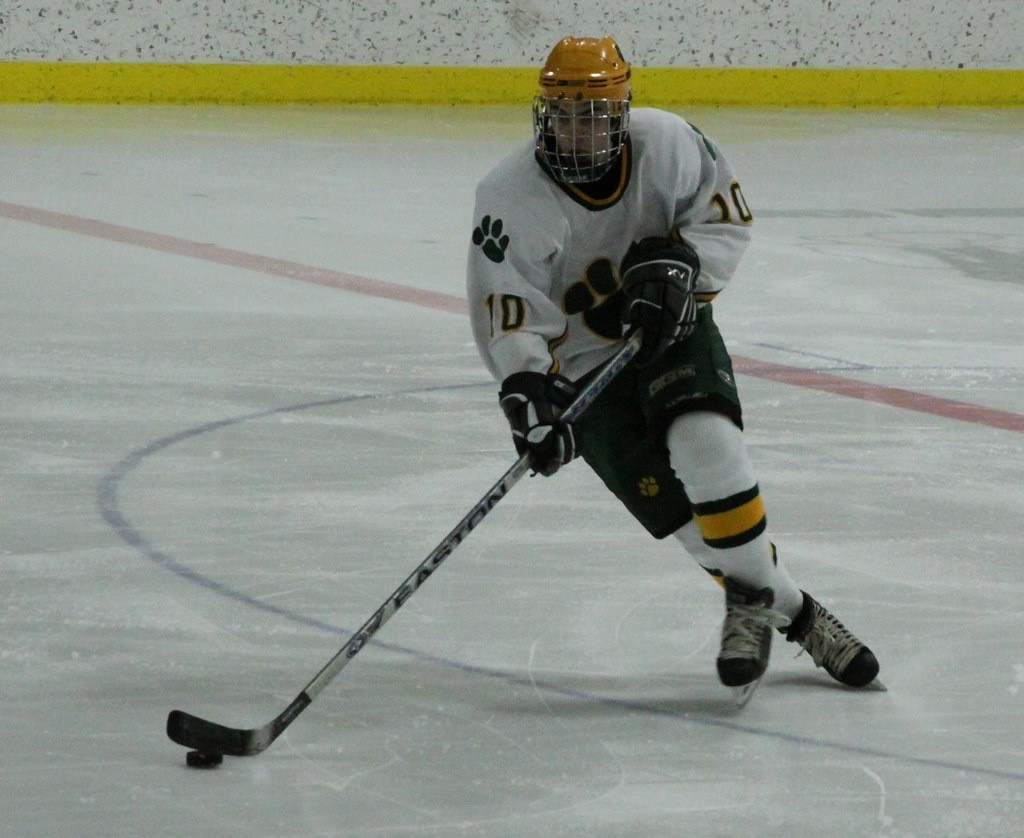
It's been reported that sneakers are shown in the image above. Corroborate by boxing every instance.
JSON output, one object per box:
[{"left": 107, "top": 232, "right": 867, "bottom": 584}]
[
  {"left": 777, "top": 590, "right": 887, "bottom": 694},
  {"left": 717, "top": 578, "right": 778, "bottom": 710}
]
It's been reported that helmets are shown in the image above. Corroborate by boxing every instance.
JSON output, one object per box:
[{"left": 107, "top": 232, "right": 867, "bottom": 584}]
[{"left": 532, "top": 36, "right": 634, "bottom": 185}]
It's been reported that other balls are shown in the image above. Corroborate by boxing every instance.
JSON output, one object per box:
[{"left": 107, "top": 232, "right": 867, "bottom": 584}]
[{"left": 186, "top": 751, "right": 222, "bottom": 769}]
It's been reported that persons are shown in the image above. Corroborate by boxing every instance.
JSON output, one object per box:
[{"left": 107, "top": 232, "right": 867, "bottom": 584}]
[{"left": 466, "top": 34, "right": 886, "bottom": 708}]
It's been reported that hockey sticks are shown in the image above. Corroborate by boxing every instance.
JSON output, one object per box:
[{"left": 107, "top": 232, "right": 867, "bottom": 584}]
[{"left": 167, "top": 329, "right": 645, "bottom": 757}]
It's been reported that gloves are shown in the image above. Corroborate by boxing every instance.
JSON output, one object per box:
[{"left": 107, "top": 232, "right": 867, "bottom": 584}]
[
  {"left": 615, "top": 236, "right": 700, "bottom": 360},
  {"left": 498, "top": 371, "right": 588, "bottom": 478}
]
